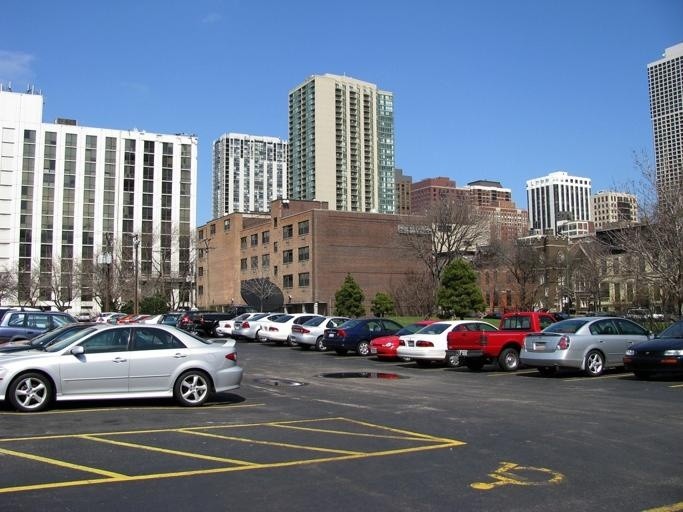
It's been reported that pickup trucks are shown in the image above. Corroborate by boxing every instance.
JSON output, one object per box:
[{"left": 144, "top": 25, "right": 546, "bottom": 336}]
[{"left": 445, "top": 312, "right": 564, "bottom": 371}]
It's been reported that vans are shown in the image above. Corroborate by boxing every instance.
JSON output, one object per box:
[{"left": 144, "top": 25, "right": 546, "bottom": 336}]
[{"left": 1, "top": 307, "right": 66, "bottom": 327}]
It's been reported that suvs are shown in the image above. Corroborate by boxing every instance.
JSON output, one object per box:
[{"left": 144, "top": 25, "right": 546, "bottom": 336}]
[{"left": 0, "top": 311, "right": 81, "bottom": 344}]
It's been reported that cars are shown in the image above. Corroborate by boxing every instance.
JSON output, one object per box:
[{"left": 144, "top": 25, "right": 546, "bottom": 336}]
[
  {"left": 323, "top": 318, "right": 412, "bottom": 358},
  {"left": 517, "top": 317, "right": 658, "bottom": 376},
  {"left": 622, "top": 320, "right": 682, "bottom": 376},
  {"left": 395, "top": 320, "right": 499, "bottom": 368},
  {"left": 0, "top": 324, "right": 244, "bottom": 413},
  {"left": 626, "top": 309, "right": 664, "bottom": 322},
  {"left": 367, "top": 320, "right": 465, "bottom": 362},
  {"left": 79, "top": 303, "right": 351, "bottom": 350}
]
